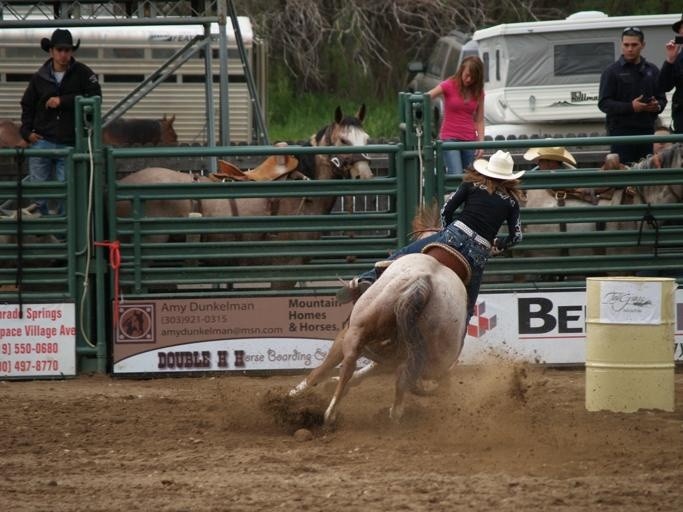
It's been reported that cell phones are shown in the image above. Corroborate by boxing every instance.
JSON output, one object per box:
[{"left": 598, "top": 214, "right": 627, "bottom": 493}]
[
  {"left": 674, "top": 35, "right": 683, "bottom": 44},
  {"left": 642, "top": 97, "right": 652, "bottom": 103}
]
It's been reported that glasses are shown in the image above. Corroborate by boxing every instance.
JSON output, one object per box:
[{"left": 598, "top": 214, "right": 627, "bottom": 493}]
[{"left": 623, "top": 27, "right": 641, "bottom": 34}]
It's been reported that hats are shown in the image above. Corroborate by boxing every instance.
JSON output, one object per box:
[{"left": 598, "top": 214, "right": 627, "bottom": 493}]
[
  {"left": 40, "top": 27, "right": 81, "bottom": 53},
  {"left": 471, "top": 148, "right": 526, "bottom": 181},
  {"left": 523, "top": 137, "right": 577, "bottom": 165},
  {"left": 671, "top": 13, "right": 683, "bottom": 34}
]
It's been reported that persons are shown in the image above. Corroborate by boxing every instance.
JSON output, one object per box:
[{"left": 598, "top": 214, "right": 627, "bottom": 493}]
[
  {"left": 521, "top": 135, "right": 577, "bottom": 171},
  {"left": 652, "top": 127, "right": 673, "bottom": 155},
  {"left": 654, "top": 14, "right": 682, "bottom": 136},
  {"left": 332, "top": 147, "right": 528, "bottom": 323},
  {"left": 18, "top": 26, "right": 103, "bottom": 218},
  {"left": 422, "top": 54, "right": 486, "bottom": 175},
  {"left": 595, "top": 26, "right": 668, "bottom": 165}
]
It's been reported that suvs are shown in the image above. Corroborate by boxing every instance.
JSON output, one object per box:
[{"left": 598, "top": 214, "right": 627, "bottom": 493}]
[{"left": 400, "top": 27, "right": 479, "bottom": 130}]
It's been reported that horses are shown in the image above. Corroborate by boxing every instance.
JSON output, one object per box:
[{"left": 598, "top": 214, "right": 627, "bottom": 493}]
[
  {"left": 101, "top": 102, "right": 373, "bottom": 290},
  {"left": 0, "top": 110, "right": 179, "bottom": 149},
  {"left": 283, "top": 194, "right": 470, "bottom": 440},
  {"left": 439, "top": 142, "right": 683, "bottom": 284}
]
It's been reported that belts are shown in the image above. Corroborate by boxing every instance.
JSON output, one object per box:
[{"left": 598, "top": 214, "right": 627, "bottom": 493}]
[{"left": 453, "top": 218, "right": 494, "bottom": 250}]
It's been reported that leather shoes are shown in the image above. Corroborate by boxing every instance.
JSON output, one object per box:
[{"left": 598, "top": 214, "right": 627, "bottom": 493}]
[{"left": 335, "top": 278, "right": 375, "bottom": 304}]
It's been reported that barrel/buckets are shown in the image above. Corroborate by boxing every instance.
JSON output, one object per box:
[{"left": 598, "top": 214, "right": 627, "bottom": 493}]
[{"left": 585, "top": 276, "right": 676, "bottom": 415}]
